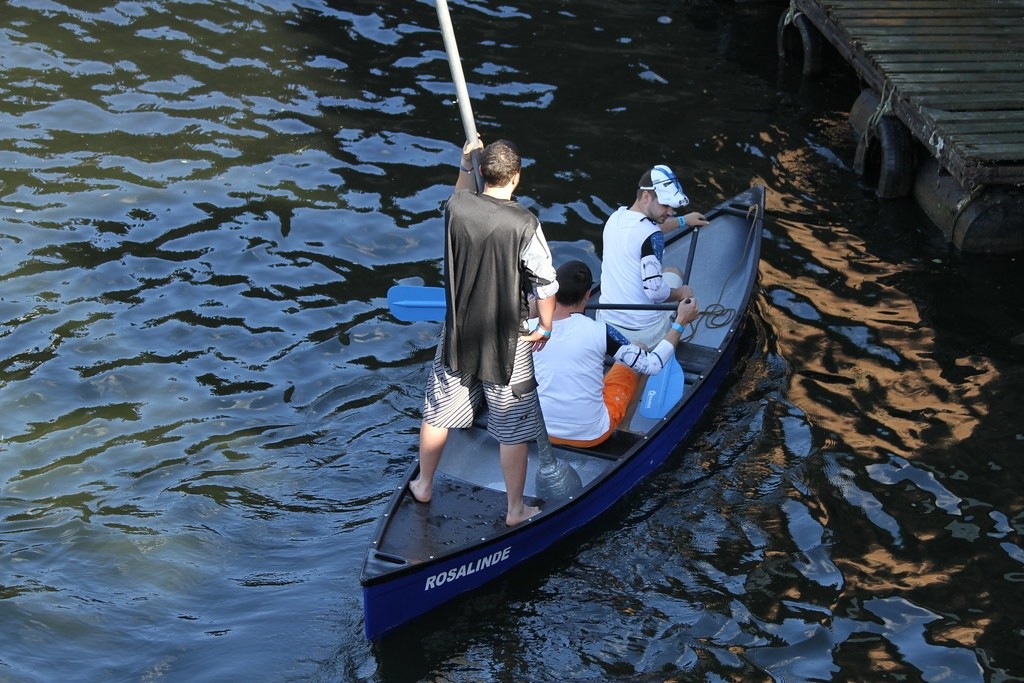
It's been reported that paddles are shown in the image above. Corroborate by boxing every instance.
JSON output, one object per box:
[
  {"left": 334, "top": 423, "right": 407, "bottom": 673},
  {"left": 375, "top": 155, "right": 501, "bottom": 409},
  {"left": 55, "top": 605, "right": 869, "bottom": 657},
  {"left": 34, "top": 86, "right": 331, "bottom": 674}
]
[
  {"left": 639, "top": 226, "right": 700, "bottom": 420},
  {"left": 387, "top": 286, "right": 692, "bottom": 323}
]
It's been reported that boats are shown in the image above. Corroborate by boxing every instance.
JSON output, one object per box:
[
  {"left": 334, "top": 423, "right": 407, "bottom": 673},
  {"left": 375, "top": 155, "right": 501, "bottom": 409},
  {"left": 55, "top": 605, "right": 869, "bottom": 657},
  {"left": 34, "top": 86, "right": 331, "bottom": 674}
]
[{"left": 358, "top": 185, "right": 765, "bottom": 638}]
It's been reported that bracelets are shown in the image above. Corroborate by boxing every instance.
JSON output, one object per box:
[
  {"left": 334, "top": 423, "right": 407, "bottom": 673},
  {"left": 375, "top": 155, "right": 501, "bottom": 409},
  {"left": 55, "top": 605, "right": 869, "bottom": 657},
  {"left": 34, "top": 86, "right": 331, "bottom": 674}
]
[
  {"left": 460, "top": 165, "right": 474, "bottom": 173},
  {"left": 536, "top": 324, "right": 551, "bottom": 336},
  {"left": 678, "top": 216, "right": 686, "bottom": 228},
  {"left": 672, "top": 323, "right": 685, "bottom": 333}
]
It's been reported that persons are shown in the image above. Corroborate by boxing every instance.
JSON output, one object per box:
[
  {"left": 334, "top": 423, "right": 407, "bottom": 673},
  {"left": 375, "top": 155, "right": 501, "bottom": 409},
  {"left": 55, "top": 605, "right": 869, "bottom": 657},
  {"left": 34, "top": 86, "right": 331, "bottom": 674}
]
[
  {"left": 529, "top": 260, "right": 700, "bottom": 448},
  {"left": 596, "top": 165, "right": 709, "bottom": 354},
  {"left": 410, "top": 133, "right": 559, "bottom": 526}
]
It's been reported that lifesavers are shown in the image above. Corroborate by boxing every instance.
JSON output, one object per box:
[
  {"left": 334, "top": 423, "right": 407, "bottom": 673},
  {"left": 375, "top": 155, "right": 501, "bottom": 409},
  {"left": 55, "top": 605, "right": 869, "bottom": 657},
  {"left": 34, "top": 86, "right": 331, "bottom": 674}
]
[
  {"left": 776, "top": 7, "right": 824, "bottom": 77},
  {"left": 853, "top": 115, "right": 917, "bottom": 198}
]
